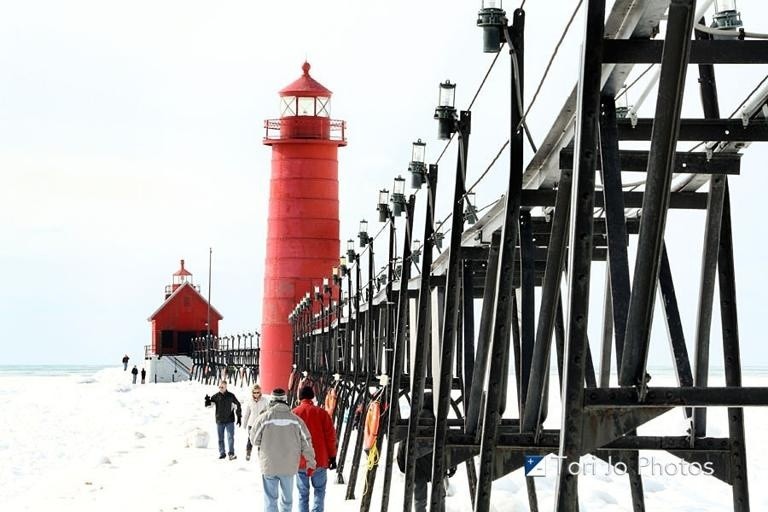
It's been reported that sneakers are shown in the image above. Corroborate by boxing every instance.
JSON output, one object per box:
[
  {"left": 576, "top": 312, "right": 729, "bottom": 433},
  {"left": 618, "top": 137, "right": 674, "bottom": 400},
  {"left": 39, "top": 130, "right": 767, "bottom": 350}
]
[{"left": 220, "top": 452, "right": 251, "bottom": 461}]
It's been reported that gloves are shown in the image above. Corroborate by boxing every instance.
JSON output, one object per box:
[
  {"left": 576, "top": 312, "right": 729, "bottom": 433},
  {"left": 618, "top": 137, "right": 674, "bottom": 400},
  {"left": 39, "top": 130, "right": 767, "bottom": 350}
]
[
  {"left": 306, "top": 467, "right": 315, "bottom": 477},
  {"left": 329, "top": 457, "right": 338, "bottom": 470},
  {"left": 237, "top": 416, "right": 242, "bottom": 427}
]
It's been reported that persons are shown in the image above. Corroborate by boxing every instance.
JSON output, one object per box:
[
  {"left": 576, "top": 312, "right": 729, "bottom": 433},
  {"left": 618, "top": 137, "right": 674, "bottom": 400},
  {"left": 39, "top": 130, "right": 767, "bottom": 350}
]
[
  {"left": 140, "top": 367, "right": 146, "bottom": 384},
  {"left": 242, "top": 383, "right": 270, "bottom": 461},
  {"left": 122, "top": 353, "right": 130, "bottom": 371},
  {"left": 397, "top": 391, "right": 457, "bottom": 511},
  {"left": 131, "top": 364, "right": 138, "bottom": 384},
  {"left": 249, "top": 387, "right": 319, "bottom": 510},
  {"left": 289, "top": 384, "right": 338, "bottom": 511},
  {"left": 205, "top": 379, "right": 242, "bottom": 462}
]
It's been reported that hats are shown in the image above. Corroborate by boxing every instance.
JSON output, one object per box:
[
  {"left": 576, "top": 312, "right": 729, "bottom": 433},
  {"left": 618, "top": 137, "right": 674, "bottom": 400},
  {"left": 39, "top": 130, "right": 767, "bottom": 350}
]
[
  {"left": 270, "top": 387, "right": 288, "bottom": 401},
  {"left": 298, "top": 386, "right": 314, "bottom": 400}
]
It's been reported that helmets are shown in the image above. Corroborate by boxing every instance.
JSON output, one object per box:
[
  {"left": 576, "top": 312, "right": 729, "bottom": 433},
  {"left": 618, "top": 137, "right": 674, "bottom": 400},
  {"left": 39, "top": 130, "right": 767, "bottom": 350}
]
[{"left": 253, "top": 392, "right": 260, "bottom": 395}]
